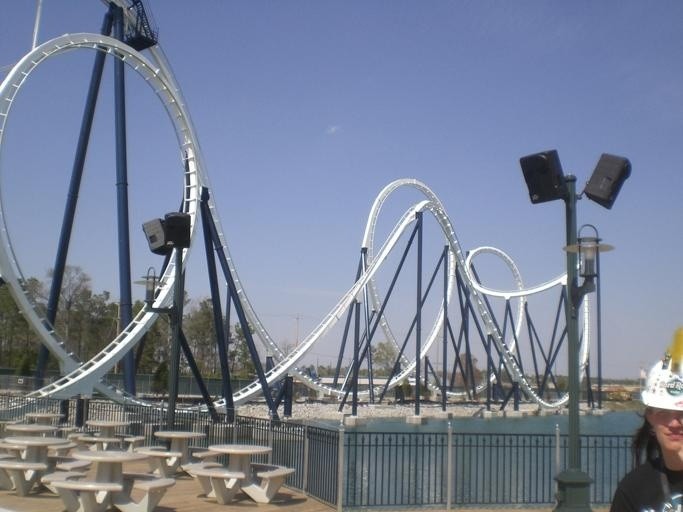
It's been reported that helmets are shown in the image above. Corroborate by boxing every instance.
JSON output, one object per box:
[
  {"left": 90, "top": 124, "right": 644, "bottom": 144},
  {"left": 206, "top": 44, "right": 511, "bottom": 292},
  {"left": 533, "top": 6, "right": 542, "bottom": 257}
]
[{"left": 641, "top": 354, "right": 683, "bottom": 411}]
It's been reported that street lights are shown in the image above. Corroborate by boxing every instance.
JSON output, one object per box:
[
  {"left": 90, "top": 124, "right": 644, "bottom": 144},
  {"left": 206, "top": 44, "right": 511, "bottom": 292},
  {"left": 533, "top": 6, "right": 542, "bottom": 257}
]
[
  {"left": 518, "top": 149, "right": 632, "bottom": 512},
  {"left": 134, "top": 211, "right": 192, "bottom": 452}
]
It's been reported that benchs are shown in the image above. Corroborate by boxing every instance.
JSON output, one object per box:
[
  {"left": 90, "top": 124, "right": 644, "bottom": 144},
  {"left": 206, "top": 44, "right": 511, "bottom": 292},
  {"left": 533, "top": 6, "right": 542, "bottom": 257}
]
[{"left": 251, "top": 463, "right": 295, "bottom": 504}]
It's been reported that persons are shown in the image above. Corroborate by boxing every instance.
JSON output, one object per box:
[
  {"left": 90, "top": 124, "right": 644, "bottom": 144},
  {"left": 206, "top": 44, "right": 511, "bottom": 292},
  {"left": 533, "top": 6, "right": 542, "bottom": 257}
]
[{"left": 608, "top": 353, "right": 682, "bottom": 511}]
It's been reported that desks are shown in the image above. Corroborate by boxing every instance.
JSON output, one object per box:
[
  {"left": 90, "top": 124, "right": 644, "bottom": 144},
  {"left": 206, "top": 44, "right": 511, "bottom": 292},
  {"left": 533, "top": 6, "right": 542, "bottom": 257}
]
[{"left": 0, "top": 414, "right": 272, "bottom": 512}]
